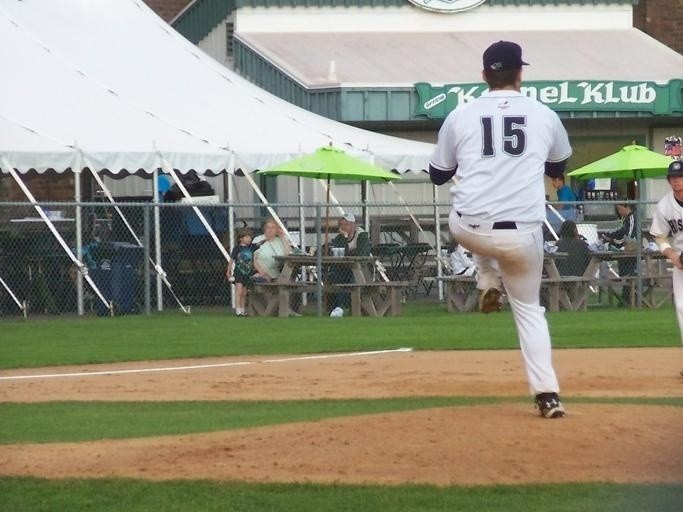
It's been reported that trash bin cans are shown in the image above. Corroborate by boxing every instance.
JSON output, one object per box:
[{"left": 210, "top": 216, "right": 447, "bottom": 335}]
[
  {"left": 44, "top": 254, "right": 77, "bottom": 313},
  {"left": 89, "top": 242, "right": 145, "bottom": 317}
]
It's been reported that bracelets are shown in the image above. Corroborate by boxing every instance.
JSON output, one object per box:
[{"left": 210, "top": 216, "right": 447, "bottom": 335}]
[{"left": 659, "top": 242, "right": 673, "bottom": 253}]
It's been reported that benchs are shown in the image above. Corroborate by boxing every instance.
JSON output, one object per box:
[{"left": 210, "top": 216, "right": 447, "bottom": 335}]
[
  {"left": 587, "top": 249, "right": 675, "bottom": 309},
  {"left": 420, "top": 248, "right": 593, "bottom": 313},
  {"left": 241, "top": 255, "right": 411, "bottom": 319}
]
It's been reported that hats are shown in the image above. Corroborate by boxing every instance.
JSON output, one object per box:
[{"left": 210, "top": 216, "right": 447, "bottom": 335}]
[
  {"left": 340, "top": 214, "right": 355, "bottom": 222},
  {"left": 667, "top": 161, "right": 683, "bottom": 176},
  {"left": 483, "top": 40, "right": 530, "bottom": 71}
]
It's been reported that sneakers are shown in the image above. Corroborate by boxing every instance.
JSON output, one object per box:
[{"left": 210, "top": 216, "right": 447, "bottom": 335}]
[
  {"left": 236, "top": 307, "right": 247, "bottom": 316},
  {"left": 478, "top": 287, "right": 503, "bottom": 313},
  {"left": 330, "top": 307, "right": 344, "bottom": 317},
  {"left": 536, "top": 393, "right": 565, "bottom": 419}
]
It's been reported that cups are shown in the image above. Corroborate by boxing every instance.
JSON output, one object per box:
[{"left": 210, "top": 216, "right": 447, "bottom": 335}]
[
  {"left": 227, "top": 276, "right": 236, "bottom": 283},
  {"left": 338, "top": 247, "right": 346, "bottom": 258},
  {"left": 332, "top": 248, "right": 339, "bottom": 257}
]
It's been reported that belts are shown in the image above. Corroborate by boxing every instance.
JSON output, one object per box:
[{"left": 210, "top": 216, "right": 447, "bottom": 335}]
[{"left": 457, "top": 210, "right": 519, "bottom": 230}]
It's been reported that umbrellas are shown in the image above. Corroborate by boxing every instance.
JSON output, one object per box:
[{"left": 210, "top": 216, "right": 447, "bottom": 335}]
[
  {"left": 567, "top": 139, "right": 679, "bottom": 309},
  {"left": 255, "top": 141, "right": 404, "bottom": 317}
]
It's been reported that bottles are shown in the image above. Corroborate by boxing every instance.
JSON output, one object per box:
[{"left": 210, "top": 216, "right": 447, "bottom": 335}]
[
  {"left": 585, "top": 189, "right": 619, "bottom": 201},
  {"left": 575, "top": 204, "right": 585, "bottom": 223}
]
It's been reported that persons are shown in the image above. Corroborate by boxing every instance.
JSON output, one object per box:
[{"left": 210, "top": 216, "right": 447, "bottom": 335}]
[
  {"left": 312, "top": 213, "right": 371, "bottom": 317},
  {"left": 252, "top": 219, "right": 295, "bottom": 284},
  {"left": 552, "top": 174, "right": 577, "bottom": 210},
  {"left": 226, "top": 232, "right": 269, "bottom": 317},
  {"left": 554, "top": 220, "right": 592, "bottom": 312},
  {"left": 649, "top": 161, "right": 683, "bottom": 377},
  {"left": 429, "top": 40, "right": 572, "bottom": 419},
  {"left": 598, "top": 198, "right": 642, "bottom": 307},
  {"left": 447, "top": 235, "right": 478, "bottom": 278}
]
